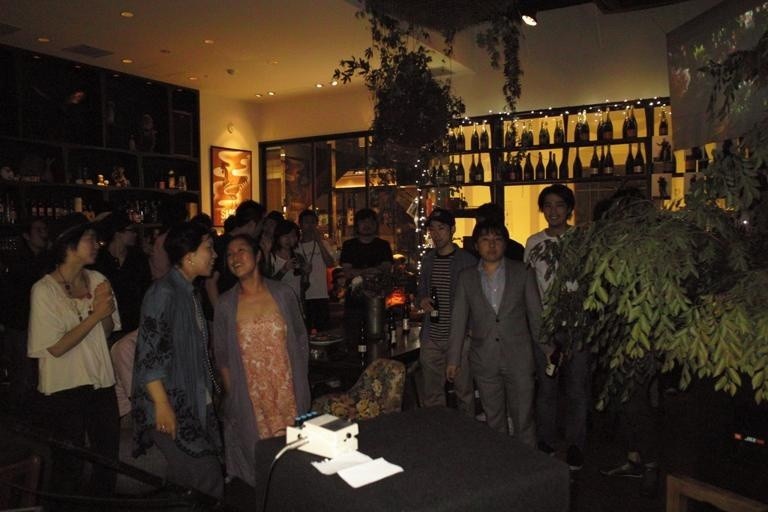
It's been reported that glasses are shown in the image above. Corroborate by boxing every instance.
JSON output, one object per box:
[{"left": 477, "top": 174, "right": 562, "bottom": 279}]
[{"left": 125, "top": 228, "right": 136, "bottom": 232}]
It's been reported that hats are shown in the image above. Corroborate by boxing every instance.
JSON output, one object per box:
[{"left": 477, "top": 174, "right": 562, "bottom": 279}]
[{"left": 425, "top": 207, "right": 455, "bottom": 226}]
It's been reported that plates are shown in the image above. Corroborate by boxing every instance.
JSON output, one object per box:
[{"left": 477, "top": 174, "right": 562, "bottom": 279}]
[{"left": 310, "top": 335, "right": 343, "bottom": 346}]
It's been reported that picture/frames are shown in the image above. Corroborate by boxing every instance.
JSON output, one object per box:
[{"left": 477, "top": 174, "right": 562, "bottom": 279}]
[{"left": 208, "top": 143, "right": 254, "bottom": 237}]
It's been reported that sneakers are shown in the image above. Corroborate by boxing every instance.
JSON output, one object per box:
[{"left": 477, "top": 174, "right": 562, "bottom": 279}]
[
  {"left": 565, "top": 446, "right": 584, "bottom": 470},
  {"left": 605, "top": 463, "right": 644, "bottom": 478},
  {"left": 536, "top": 441, "right": 554, "bottom": 454}
]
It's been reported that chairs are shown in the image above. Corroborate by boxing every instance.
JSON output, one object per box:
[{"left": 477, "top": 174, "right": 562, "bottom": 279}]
[{"left": 312, "top": 360, "right": 408, "bottom": 430}]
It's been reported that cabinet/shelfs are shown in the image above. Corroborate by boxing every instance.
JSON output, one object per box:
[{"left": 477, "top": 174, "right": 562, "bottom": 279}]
[
  {"left": 0, "top": 42, "right": 201, "bottom": 248},
  {"left": 498, "top": 98, "right": 653, "bottom": 185},
  {"left": 424, "top": 115, "right": 503, "bottom": 218}
]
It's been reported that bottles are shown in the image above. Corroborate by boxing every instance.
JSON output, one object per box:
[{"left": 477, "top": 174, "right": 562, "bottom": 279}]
[
  {"left": 357, "top": 328, "right": 368, "bottom": 366},
  {"left": 431, "top": 122, "right": 490, "bottom": 183},
  {"left": 28, "top": 189, "right": 95, "bottom": 222},
  {"left": 389, "top": 311, "right": 397, "bottom": 346},
  {"left": 501, "top": 107, "right": 709, "bottom": 179},
  {"left": 157, "top": 170, "right": 187, "bottom": 191},
  {"left": 430, "top": 286, "right": 440, "bottom": 323},
  {"left": 402, "top": 301, "right": 411, "bottom": 334}
]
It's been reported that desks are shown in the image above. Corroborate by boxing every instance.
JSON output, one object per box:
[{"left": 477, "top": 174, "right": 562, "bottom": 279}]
[
  {"left": 251, "top": 412, "right": 572, "bottom": 511},
  {"left": 663, "top": 451, "right": 766, "bottom": 512}
]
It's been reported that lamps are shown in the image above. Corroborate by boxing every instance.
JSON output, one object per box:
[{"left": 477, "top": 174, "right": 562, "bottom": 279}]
[{"left": 520, "top": 6, "right": 538, "bottom": 28}]
